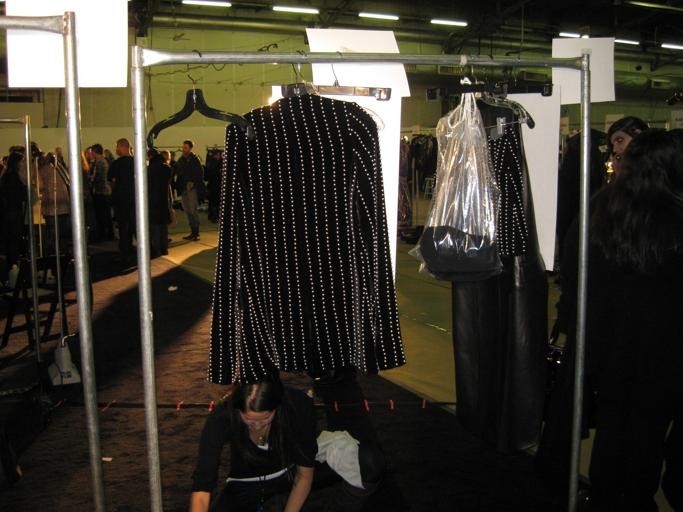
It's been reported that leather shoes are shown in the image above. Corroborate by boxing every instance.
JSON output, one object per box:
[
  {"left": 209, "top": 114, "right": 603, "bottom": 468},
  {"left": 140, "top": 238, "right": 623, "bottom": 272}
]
[{"left": 183, "top": 235, "right": 200, "bottom": 241}]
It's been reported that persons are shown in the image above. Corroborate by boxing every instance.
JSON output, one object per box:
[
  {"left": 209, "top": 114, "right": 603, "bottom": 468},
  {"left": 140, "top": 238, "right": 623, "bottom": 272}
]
[
  {"left": 185, "top": 381, "right": 319, "bottom": 511},
  {"left": 0, "top": 138, "right": 223, "bottom": 295},
  {"left": 608, "top": 117, "right": 647, "bottom": 159},
  {"left": 532, "top": 128, "right": 682, "bottom": 512}
]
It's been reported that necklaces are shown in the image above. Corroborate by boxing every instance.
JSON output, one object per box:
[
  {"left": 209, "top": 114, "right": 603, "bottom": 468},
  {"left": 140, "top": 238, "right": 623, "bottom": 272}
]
[
  {"left": 251, "top": 426, "right": 269, "bottom": 446},
  {"left": 251, "top": 448, "right": 269, "bottom": 503}
]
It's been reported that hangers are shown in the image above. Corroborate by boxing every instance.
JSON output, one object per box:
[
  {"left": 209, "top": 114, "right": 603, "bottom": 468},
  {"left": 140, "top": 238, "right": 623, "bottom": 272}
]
[
  {"left": 281, "top": 51, "right": 391, "bottom": 100},
  {"left": 447, "top": 53, "right": 535, "bottom": 130},
  {"left": 147, "top": 50, "right": 253, "bottom": 147},
  {"left": 289, "top": 49, "right": 306, "bottom": 95}
]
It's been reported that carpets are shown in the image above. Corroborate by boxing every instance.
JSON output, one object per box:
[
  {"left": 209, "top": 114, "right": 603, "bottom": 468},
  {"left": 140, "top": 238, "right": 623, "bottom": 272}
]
[{"left": 0, "top": 259, "right": 654, "bottom": 512}]
